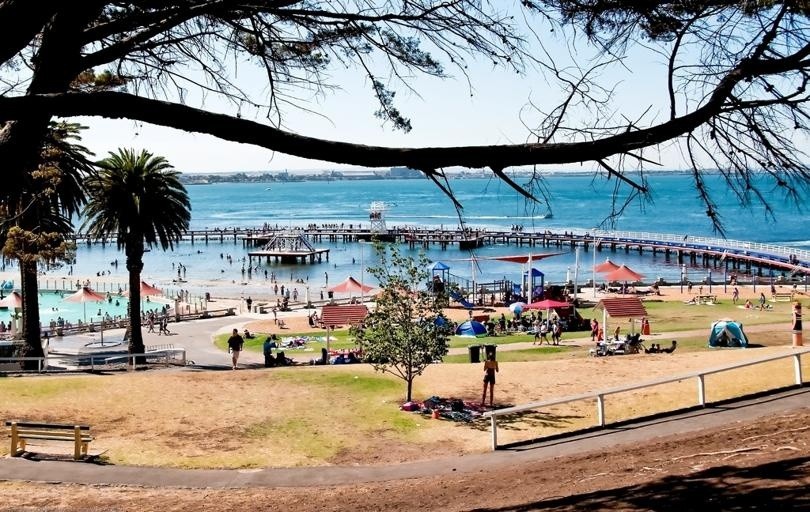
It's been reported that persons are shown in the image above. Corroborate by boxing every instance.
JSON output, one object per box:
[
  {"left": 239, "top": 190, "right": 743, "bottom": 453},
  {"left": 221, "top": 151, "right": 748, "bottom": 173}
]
[
  {"left": 729, "top": 272, "right": 737, "bottom": 286},
  {"left": 277, "top": 351, "right": 300, "bottom": 365},
  {"left": 759, "top": 300, "right": 770, "bottom": 309},
  {"left": 301, "top": 348, "right": 330, "bottom": 365},
  {"left": 50, "top": 318, "right": 56, "bottom": 335},
  {"left": 227, "top": 328, "right": 245, "bottom": 371},
  {"left": 769, "top": 284, "right": 776, "bottom": 303},
  {"left": 0, "top": 320, "right": 7, "bottom": 332},
  {"left": 717, "top": 331, "right": 731, "bottom": 346},
  {"left": 8, "top": 321, "right": 12, "bottom": 331},
  {"left": 347, "top": 352, "right": 354, "bottom": 358},
  {"left": 66, "top": 287, "right": 189, "bottom": 335},
  {"left": 731, "top": 287, "right": 739, "bottom": 306},
  {"left": 430, "top": 274, "right": 677, "bottom": 358},
  {"left": 480, "top": 352, "right": 499, "bottom": 409},
  {"left": 758, "top": 293, "right": 767, "bottom": 311},
  {"left": 332, "top": 354, "right": 344, "bottom": 364},
  {"left": 263, "top": 336, "right": 278, "bottom": 367},
  {"left": 57, "top": 317, "right": 64, "bottom": 336},
  {"left": 172, "top": 251, "right": 330, "bottom": 340},
  {"left": 744, "top": 299, "right": 760, "bottom": 310}
]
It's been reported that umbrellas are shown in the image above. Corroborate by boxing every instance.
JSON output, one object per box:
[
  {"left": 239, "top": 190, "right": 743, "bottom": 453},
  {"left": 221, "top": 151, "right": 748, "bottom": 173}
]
[
  {"left": 0, "top": 289, "right": 22, "bottom": 308},
  {"left": 603, "top": 262, "right": 646, "bottom": 297},
  {"left": 594, "top": 256, "right": 621, "bottom": 273},
  {"left": 329, "top": 275, "right": 373, "bottom": 305},
  {"left": 64, "top": 286, "right": 106, "bottom": 323},
  {"left": 121, "top": 278, "right": 163, "bottom": 312}
]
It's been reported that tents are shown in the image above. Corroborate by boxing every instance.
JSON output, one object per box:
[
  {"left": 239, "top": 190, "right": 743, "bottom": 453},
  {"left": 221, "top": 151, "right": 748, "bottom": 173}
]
[{"left": 708, "top": 317, "right": 749, "bottom": 349}]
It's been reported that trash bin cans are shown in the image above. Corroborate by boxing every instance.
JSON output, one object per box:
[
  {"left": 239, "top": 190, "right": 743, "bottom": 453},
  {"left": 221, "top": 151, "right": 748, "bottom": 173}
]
[
  {"left": 483, "top": 344, "right": 496, "bottom": 361},
  {"left": 468, "top": 345, "right": 481, "bottom": 363}
]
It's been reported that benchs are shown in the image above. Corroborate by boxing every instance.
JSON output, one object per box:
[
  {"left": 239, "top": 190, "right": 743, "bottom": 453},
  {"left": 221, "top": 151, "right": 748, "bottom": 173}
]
[{"left": 4, "top": 420, "right": 91, "bottom": 459}]
